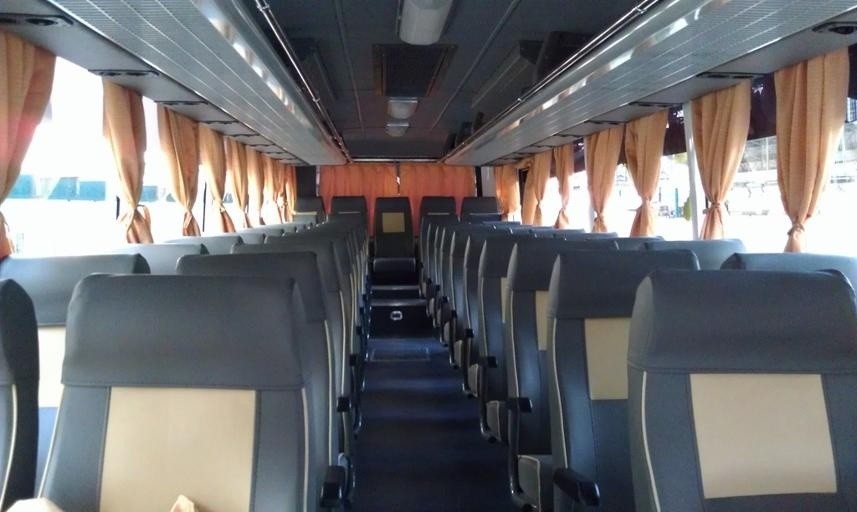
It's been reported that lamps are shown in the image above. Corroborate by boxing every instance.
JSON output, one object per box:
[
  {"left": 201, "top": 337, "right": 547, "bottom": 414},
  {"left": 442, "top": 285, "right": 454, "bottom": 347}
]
[{"left": 385, "top": 1, "right": 454, "bottom": 138}]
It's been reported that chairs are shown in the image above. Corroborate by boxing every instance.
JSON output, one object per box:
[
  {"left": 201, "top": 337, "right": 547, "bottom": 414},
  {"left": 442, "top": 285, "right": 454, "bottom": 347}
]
[
  {"left": 422, "top": 192, "right": 856, "bottom": 509},
  {"left": 374, "top": 196, "right": 418, "bottom": 274},
  {"left": 1, "top": 194, "right": 371, "bottom": 512}
]
